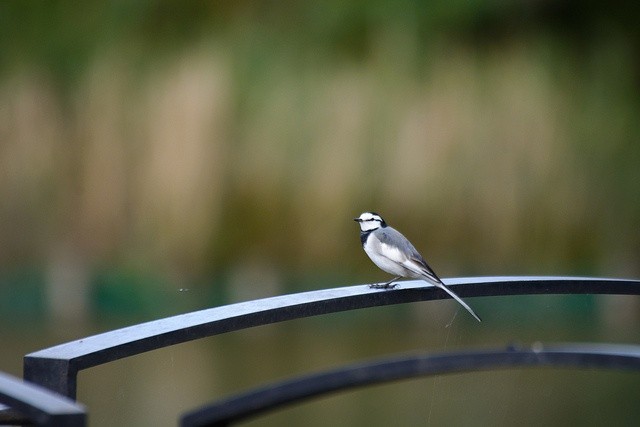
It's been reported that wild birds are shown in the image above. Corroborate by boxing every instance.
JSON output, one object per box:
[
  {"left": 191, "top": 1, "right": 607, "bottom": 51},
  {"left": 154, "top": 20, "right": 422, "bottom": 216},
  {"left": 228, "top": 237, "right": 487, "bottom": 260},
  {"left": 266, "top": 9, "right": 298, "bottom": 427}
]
[{"left": 353, "top": 210, "right": 483, "bottom": 324}]
